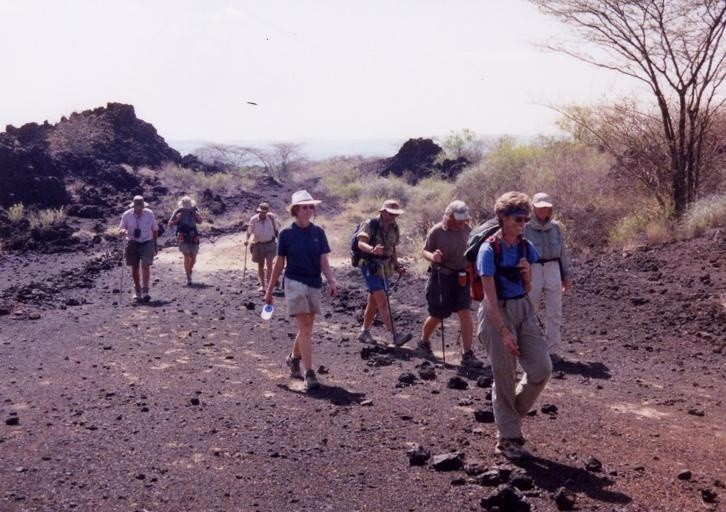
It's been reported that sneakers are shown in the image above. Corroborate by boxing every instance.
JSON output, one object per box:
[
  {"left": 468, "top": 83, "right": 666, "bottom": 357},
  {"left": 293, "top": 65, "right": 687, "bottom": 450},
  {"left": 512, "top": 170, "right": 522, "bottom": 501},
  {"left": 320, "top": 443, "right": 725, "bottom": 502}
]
[{"left": 495, "top": 439, "right": 529, "bottom": 461}]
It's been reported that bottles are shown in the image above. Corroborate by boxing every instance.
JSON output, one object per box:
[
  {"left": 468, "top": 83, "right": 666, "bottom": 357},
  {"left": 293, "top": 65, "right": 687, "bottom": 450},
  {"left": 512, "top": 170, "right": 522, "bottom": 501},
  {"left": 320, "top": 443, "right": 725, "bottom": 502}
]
[
  {"left": 458, "top": 272, "right": 466, "bottom": 286},
  {"left": 178, "top": 232, "right": 184, "bottom": 242},
  {"left": 261, "top": 304, "right": 275, "bottom": 320}
]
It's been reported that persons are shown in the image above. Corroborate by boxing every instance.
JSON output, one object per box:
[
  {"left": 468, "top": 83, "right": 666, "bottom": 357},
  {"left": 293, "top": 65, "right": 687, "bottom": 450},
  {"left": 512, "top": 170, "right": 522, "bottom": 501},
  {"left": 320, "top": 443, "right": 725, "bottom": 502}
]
[
  {"left": 520, "top": 193, "right": 573, "bottom": 364},
  {"left": 168, "top": 195, "right": 203, "bottom": 285},
  {"left": 465, "top": 190, "right": 553, "bottom": 459},
  {"left": 117, "top": 194, "right": 159, "bottom": 303},
  {"left": 414, "top": 199, "right": 484, "bottom": 369},
  {"left": 356, "top": 198, "right": 413, "bottom": 348},
  {"left": 263, "top": 190, "right": 340, "bottom": 391},
  {"left": 243, "top": 202, "right": 282, "bottom": 292}
]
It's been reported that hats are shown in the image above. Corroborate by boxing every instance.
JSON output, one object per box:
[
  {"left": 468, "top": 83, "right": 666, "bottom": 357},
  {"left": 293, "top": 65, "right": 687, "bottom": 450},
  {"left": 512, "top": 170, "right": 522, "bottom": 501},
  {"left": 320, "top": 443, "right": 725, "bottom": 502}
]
[
  {"left": 178, "top": 197, "right": 195, "bottom": 209},
  {"left": 128, "top": 196, "right": 148, "bottom": 208},
  {"left": 532, "top": 193, "right": 552, "bottom": 208},
  {"left": 287, "top": 190, "right": 321, "bottom": 212},
  {"left": 257, "top": 203, "right": 268, "bottom": 213},
  {"left": 442, "top": 200, "right": 469, "bottom": 231},
  {"left": 379, "top": 201, "right": 403, "bottom": 215}
]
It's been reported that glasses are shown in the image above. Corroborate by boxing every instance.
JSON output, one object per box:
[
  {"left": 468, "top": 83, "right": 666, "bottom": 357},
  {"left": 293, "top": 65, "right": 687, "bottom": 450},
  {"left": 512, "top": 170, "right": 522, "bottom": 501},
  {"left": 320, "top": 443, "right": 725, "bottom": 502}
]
[{"left": 514, "top": 216, "right": 531, "bottom": 222}]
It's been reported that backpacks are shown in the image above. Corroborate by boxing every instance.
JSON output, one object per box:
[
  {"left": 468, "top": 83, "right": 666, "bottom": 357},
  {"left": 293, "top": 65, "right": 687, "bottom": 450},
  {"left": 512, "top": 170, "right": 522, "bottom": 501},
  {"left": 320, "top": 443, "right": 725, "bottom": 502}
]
[
  {"left": 464, "top": 219, "right": 502, "bottom": 301},
  {"left": 351, "top": 219, "right": 378, "bottom": 266}
]
